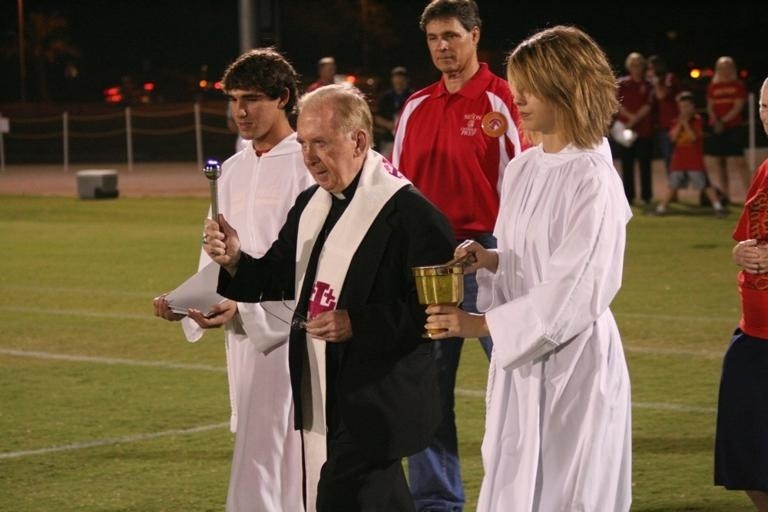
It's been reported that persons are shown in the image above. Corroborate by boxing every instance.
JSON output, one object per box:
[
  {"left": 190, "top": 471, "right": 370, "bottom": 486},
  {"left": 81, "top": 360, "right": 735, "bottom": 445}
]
[
  {"left": 390, "top": 1, "right": 541, "bottom": 512},
  {"left": 593, "top": 48, "right": 751, "bottom": 217},
  {"left": 305, "top": 57, "right": 336, "bottom": 95},
  {"left": 200, "top": 82, "right": 471, "bottom": 511},
  {"left": 426, "top": 27, "right": 635, "bottom": 512},
  {"left": 151, "top": 49, "right": 330, "bottom": 509},
  {"left": 373, "top": 67, "right": 419, "bottom": 142},
  {"left": 712, "top": 78, "right": 767, "bottom": 511}
]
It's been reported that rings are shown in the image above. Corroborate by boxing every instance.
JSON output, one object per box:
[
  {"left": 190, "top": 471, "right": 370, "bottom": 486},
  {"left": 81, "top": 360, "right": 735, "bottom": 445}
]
[
  {"left": 199, "top": 235, "right": 208, "bottom": 242},
  {"left": 756, "top": 262, "right": 761, "bottom": 270}
]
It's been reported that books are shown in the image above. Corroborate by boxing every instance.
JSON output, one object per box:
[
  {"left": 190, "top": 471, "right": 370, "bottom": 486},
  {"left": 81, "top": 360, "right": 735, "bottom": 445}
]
[{"left": 164, "top": 260, "right": 229, "bottom": 318}]
